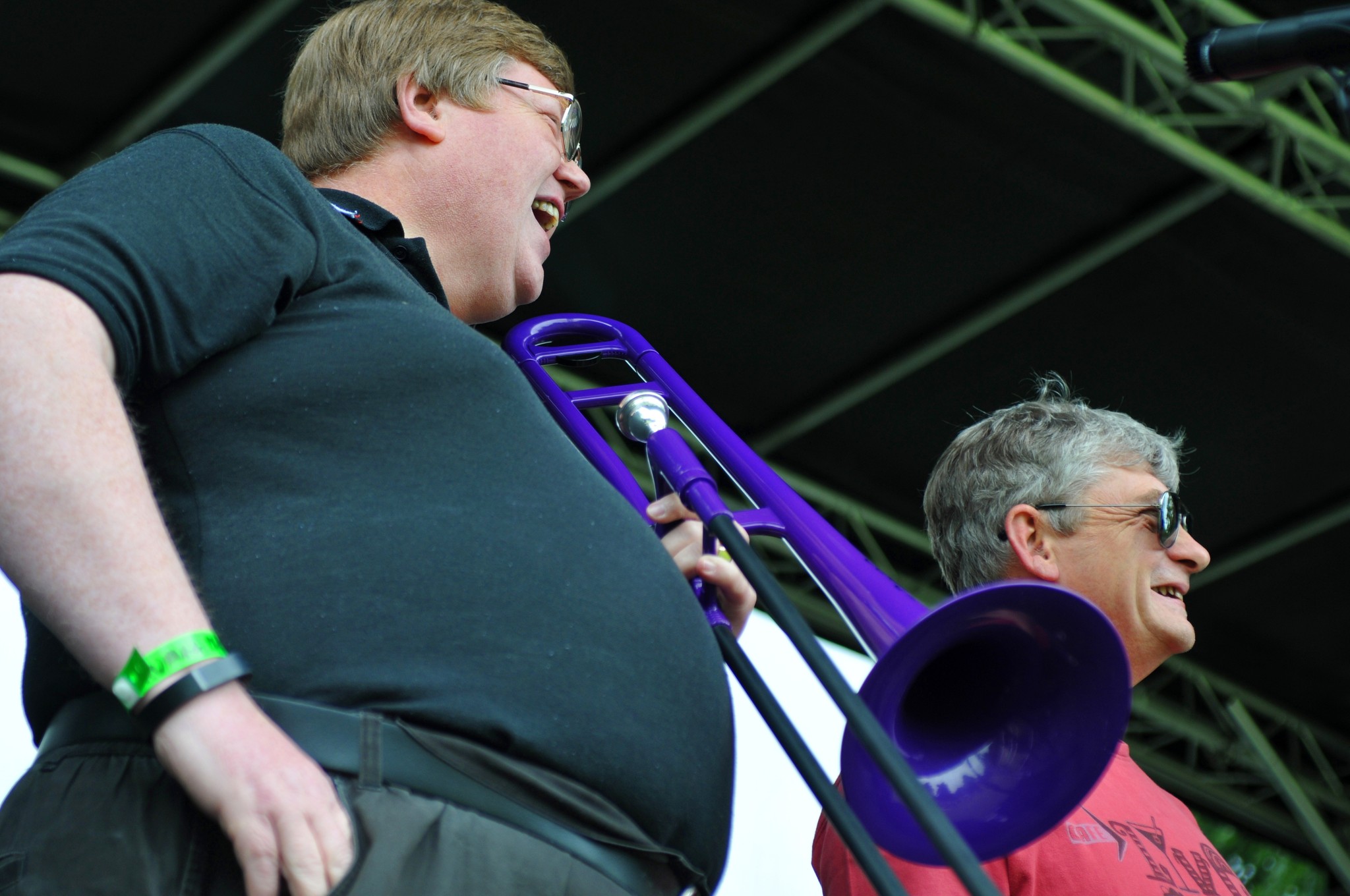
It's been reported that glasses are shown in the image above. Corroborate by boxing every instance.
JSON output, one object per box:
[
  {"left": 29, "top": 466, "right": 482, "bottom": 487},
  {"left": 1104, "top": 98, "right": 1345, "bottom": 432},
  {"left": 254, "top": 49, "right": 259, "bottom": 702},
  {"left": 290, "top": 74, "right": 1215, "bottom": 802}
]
[
  {"left": 484, "top": 73, "right": 582, "bottom": 170},
  {"left": 996, "top": 493, "right": 1189, "bottom": 549}
]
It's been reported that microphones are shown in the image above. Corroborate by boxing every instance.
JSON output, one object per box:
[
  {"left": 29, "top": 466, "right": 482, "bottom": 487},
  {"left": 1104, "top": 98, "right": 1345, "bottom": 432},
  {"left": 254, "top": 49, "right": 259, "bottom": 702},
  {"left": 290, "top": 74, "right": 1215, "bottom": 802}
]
[{"left": 1186, "top": 5, "right": 1350, "bottom": 82}]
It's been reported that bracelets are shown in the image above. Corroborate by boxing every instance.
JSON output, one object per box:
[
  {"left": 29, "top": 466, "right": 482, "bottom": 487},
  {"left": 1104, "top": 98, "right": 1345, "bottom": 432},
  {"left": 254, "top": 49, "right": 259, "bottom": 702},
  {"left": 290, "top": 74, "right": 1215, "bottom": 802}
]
[
  {"left": 129, "top": 651, "right": 253, "bottom": 745},
  {"left": 108, "top": 627, "right": 228, "bottom": 711}
]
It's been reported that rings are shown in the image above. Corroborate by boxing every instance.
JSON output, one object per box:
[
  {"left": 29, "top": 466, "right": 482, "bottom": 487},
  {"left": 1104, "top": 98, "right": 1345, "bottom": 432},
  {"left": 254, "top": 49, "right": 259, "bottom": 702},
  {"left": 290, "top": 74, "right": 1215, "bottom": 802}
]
[{"left": 718, "top": 540, "right": 734, "bottom": 563}]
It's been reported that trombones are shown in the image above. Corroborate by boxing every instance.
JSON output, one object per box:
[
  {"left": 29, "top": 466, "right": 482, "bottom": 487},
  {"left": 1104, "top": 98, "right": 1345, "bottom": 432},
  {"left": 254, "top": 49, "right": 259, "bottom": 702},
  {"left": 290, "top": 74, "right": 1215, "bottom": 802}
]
[{"left": 510, "top": 312, "right": 1132, "bottom": 896}]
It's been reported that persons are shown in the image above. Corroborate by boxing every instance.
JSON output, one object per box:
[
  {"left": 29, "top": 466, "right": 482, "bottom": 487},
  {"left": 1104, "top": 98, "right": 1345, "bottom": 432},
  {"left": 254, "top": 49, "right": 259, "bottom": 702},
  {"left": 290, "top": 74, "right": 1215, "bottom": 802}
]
[
  {"left": 0, "top": 1, "right": 760, "bottom": 895},
  {"left": 804, "top": 374, "right": 1256, "bottom": 896}
]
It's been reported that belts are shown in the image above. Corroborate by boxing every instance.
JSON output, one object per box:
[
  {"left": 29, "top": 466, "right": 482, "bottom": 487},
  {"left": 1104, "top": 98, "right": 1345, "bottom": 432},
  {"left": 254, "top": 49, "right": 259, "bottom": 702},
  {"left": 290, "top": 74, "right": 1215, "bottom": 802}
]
[{"left": 40, "top": 687, "right": 698, "bottom": 896}]
